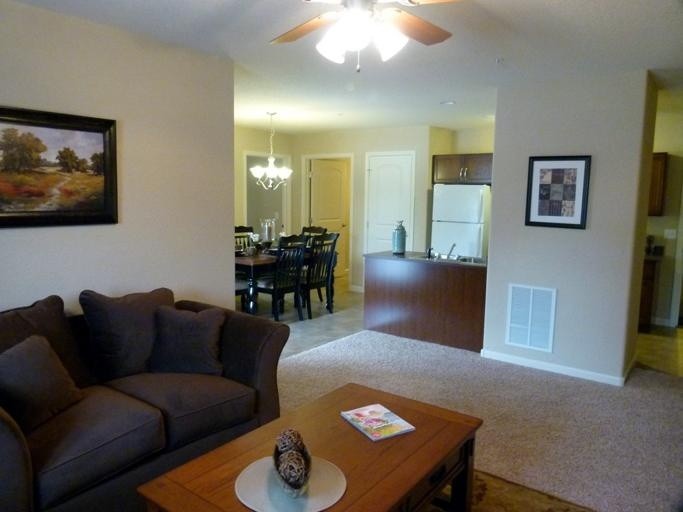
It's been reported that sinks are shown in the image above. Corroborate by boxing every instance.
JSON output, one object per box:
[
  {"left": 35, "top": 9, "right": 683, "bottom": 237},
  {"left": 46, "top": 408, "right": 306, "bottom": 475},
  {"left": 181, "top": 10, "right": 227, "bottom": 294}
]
[
  {"left": 421, "top": 254, "right": 455, "bottom": 262},
  {"left": 459, "top": 258, "right": 483, "bottom": 263}
]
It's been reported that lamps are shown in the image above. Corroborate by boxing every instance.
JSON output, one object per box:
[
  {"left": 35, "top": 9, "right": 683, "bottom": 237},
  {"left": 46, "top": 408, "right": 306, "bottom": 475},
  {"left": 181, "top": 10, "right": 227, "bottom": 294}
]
[
  {"left": 311, "top": 14, "right": 410, "bottom": 64},
  {"left": 249, "top": 107, "right": 292, "bottom": 192}
]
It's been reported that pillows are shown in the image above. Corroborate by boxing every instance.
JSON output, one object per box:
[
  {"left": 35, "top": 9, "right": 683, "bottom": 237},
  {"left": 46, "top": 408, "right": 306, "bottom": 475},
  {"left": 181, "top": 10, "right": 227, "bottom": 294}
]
[
  {"left": 78, "top": 286, "right": 174, "bottom": 379},
  {"left": 0, "top": 290, "right": 102, "bottom": 389},
  {"left": 2, "top": 332, "right": 84, "bottom": 432},
  {"left": 154, "top": 302, "right": 225, "bottom": 379}
]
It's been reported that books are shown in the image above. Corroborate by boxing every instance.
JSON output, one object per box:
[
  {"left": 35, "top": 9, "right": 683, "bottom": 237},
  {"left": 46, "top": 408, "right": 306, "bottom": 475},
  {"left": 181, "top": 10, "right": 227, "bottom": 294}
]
[{"left": 340, "top": 403, "right": 416, "bottom": 442}]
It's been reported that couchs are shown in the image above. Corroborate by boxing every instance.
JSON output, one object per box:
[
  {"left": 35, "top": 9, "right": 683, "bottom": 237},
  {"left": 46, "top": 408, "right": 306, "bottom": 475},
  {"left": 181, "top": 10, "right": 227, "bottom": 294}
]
[{"left": 0, "top": 282, "right": 292, "bottom": 511}]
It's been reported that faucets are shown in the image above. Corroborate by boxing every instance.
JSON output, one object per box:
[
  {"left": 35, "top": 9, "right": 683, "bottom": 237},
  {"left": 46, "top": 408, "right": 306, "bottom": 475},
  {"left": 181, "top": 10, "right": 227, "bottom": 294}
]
[{"left": 447, "top": 243, "right": 456, "bottom": 261}]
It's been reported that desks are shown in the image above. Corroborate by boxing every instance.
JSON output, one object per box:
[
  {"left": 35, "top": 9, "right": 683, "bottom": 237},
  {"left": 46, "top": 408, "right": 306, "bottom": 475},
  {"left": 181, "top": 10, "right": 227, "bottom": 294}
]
[
  {"left": 360, "top": 249, "right": 488, "bottom": 353},
  {"left": 638, "top": 254, "right": 662, "bottom": 333}
]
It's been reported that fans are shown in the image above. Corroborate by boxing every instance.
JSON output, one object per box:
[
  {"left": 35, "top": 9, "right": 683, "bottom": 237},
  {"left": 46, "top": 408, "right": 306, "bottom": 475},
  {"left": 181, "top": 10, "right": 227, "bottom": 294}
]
[{"left": 268, "top": 0, "right": 467, "bottom": 47}]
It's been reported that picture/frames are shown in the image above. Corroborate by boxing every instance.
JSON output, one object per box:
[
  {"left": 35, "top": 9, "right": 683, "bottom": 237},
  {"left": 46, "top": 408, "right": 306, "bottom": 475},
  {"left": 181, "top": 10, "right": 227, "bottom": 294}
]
[
  {"left": 524, "top": 154, "right": 591, "bottom": 230},
  {"left": 0, "top": 105, "right": 119, "bottom": 230}
]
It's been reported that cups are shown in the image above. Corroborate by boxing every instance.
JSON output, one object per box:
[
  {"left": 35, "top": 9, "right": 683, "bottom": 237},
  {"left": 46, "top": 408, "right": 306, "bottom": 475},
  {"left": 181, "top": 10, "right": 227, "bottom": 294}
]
[{"left": 246, "top": 247, "right": 255, "bottom": 256}]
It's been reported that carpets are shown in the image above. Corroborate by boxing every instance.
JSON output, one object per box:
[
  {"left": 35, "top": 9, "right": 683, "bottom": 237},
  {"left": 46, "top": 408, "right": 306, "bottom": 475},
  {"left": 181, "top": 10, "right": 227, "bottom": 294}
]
[
  {"left": 418, "top": 464, "right": 596, "bottom": 512},
  {"left": 275, "top": 329, "right": 683, "bottom": 510}
]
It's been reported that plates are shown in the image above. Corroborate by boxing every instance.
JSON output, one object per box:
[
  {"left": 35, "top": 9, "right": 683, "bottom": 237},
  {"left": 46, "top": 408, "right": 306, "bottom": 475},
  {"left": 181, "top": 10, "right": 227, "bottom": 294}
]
[
  {"left": 235, "top": 247, "right": 243, "bottom": 255},
  {"left": 266, "top": 245, "right": 310, "bottom": 252}
]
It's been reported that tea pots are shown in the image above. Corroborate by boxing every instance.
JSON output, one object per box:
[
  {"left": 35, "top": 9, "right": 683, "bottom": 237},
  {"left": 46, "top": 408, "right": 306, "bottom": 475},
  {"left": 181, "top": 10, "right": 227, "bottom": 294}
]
[{"left": 258, "top": 215, "right": 276, "bottom": 242}]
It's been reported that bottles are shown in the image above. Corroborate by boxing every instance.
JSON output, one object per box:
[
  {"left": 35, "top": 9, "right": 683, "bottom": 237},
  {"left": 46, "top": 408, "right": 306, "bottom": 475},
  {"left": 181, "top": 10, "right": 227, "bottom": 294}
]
[
  {"left": 392, "top": 219, "right": 407, "bottom": 256},
  {"left": 278, "top": 224, "right": 287, "bottom": 240}
]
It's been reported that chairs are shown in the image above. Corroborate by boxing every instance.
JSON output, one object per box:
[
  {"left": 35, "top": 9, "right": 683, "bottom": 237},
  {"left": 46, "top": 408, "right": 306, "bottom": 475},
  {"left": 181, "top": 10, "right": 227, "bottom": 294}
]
[{"left": 235, "top": 224, "right": 340, "bottom": 322}]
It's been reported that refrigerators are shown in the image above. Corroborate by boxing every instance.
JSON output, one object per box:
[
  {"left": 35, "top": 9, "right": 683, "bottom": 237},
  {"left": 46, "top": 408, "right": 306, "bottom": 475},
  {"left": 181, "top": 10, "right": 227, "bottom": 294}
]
[{"left": 430, "top": 182, "right": 491, "bottom": 258}]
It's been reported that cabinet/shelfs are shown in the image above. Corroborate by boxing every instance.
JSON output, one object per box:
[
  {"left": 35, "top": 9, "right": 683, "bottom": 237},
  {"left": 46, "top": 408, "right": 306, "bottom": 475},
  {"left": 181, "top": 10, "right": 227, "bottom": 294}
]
[
  {"left": 432, "top": 152, "right": 492, "bottom": 185},
  {"left": 646, "top": 152, "right": 668, "bottom": 217}
]
